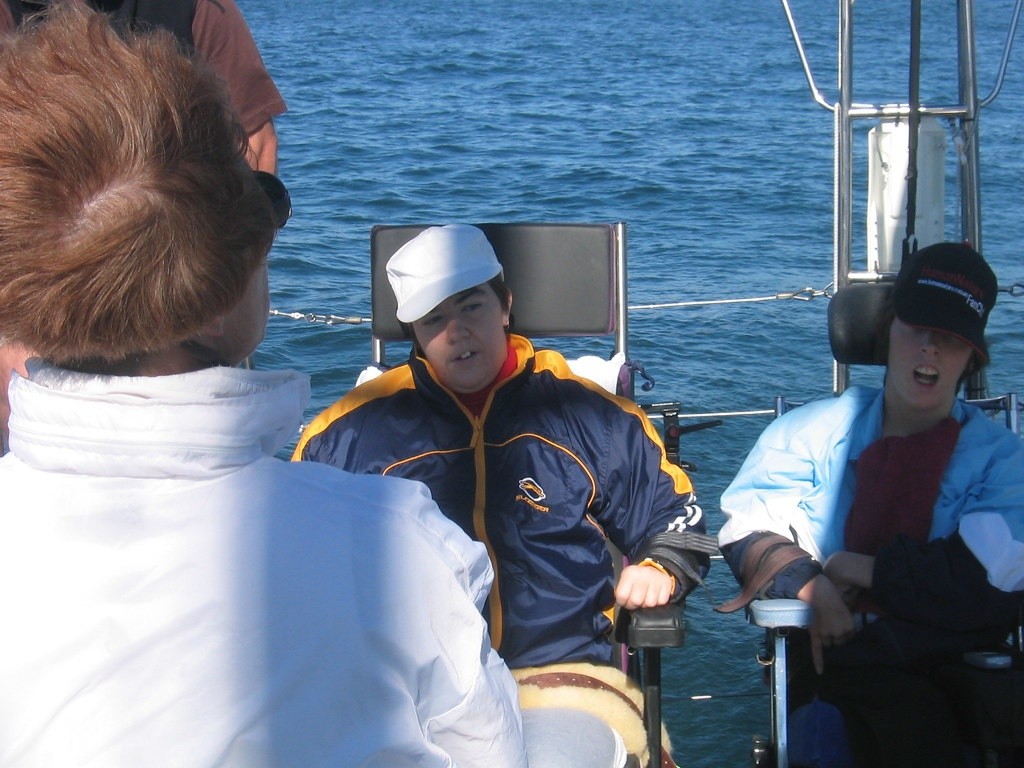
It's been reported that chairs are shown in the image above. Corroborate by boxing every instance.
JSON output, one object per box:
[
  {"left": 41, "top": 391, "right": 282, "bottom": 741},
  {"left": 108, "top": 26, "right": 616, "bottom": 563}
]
[
  {"left": 748, "top": 282, "right": 1024, "bottom": 768},
  {"left": 354, "top": 222, "right": 684, "bottom": 768}
]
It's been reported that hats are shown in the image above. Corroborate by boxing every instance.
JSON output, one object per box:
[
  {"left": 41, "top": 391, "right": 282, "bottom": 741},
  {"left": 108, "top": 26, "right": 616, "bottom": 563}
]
[
  {"left": 386, "top": 223, "right": 505, "bottom": 323},
  {"left": 892, "top": 241, "right": 998, "bottom": 363}
]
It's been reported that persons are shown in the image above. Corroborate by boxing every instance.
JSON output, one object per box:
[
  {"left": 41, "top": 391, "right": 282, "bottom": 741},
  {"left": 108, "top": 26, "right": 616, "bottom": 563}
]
[
  {"left": 288, "top": 223, "right": 710, "bottom": 768},
  {"left": 716, "top": 242, "right": 1024, "bottom": 768},
  {"left": 0, "top": 0, "right": 631, "bottom": 768}
]
